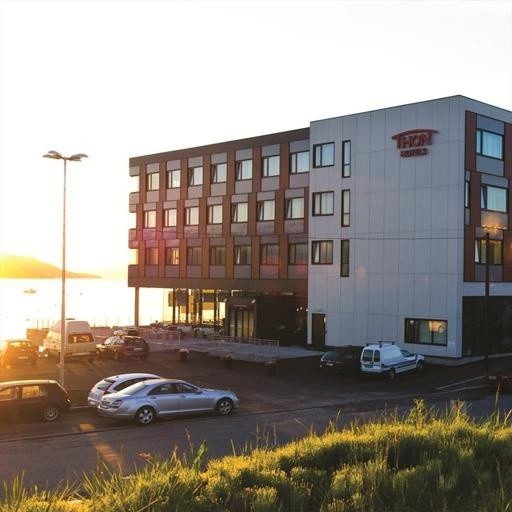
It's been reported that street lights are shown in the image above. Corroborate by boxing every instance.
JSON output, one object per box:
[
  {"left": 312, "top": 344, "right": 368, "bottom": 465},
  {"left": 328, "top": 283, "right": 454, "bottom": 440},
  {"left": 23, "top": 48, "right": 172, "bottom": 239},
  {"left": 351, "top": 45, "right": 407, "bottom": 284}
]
[{"left": 42, "top": 150, "right": 88, "bottom": 387}]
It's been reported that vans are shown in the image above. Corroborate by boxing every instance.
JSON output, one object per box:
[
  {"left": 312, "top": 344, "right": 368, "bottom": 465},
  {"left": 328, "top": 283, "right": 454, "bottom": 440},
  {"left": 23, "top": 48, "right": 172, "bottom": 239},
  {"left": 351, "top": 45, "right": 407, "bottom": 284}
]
[
  {"left": 44, "top": 320, "right": 96, "bottom": 363},
  {"left": 360, "top": 343, "right": 425, "bottom": 381}
]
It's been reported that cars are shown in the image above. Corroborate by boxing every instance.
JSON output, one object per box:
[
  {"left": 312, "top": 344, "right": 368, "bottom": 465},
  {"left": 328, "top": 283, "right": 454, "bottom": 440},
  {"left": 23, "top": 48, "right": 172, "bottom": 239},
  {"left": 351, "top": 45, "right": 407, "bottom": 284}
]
[
  {"left": 0, "top": 380, "right": 72, "bottom": 422},
  {"left": 4, "top": 339, "right": 37, "bottom": 366},
  {"left": 88, "top": 373, "right": 240, "bottom": 425},
  {"left": 96, "top": 335, "right": 150, "bottom": 361},
  {"left": 320, "top": 346, "right": 365, "bottom": 375},
  {"left": 488, "top": 359, "right": 512, "bottom": 394},
  {"left": 113, "top": 330, "right": 139, "bottom": 335}
]
